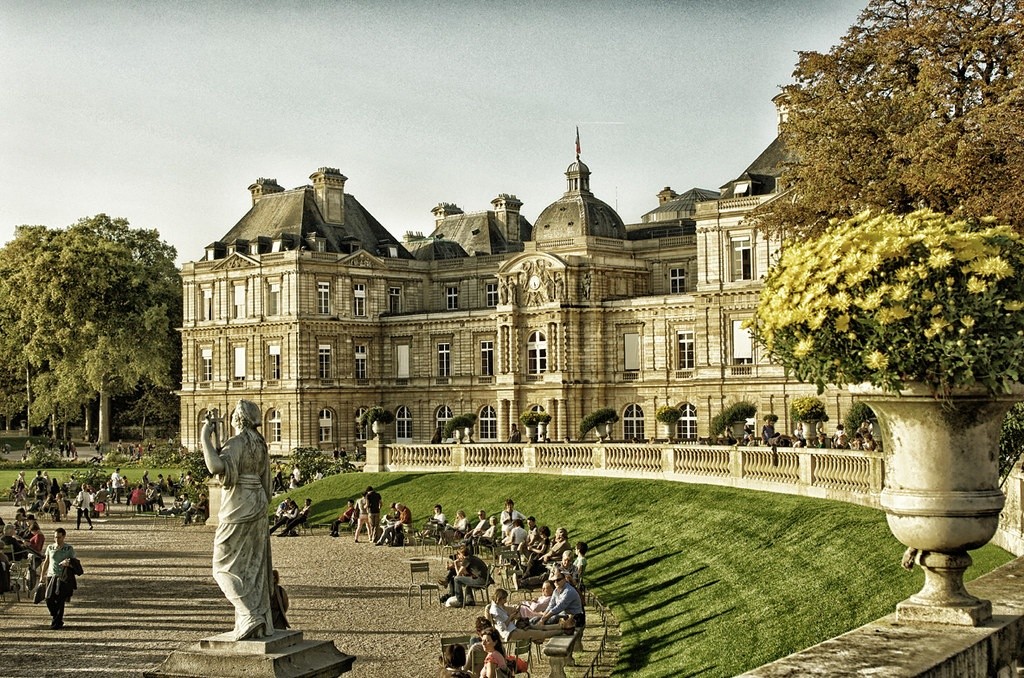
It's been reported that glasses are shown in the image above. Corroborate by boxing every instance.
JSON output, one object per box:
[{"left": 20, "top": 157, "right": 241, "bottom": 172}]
[
  {"left": 478, "top": 514, "right": 484, "bottom": 516},
  {"left": 553, "top": 578, "right": 564, "bottom": 583}
]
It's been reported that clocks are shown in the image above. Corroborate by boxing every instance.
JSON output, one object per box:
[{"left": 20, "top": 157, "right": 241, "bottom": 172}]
[{"left": 529, "top": 275, "right": 540, "bottom": 289}]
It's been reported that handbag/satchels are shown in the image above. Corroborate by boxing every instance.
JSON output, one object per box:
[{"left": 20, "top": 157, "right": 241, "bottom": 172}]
[
  {"left": 516, "top": 617, "right": 530, "bottom": 629},
  {"left": 33, "top": 585, "right": 46, "bottom": 604},
  {"left": 76, "top": 500, "right": 83, "bottom": 509}
]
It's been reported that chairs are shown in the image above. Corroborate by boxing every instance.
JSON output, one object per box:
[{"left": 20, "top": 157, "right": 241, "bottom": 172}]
[
  {"left": 401, "top": 523, "right": 544, "bottom": 678},
  {"left": 0, "top": 477, "right": 315, "bottom": 603}
]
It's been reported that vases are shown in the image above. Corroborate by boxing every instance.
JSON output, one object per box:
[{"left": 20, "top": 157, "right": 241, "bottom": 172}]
[
  {"left": 801, "top": 421, "right": 818, "bottom": 437},
  {"left": 847, "top": 381, "right": 1024, "bottom": 553},
  {"left": 525, "top": 422, "right": 547, "bottom": 438},
  {"left": 663, "top": 423, "right": 677, "bottom": 437}
]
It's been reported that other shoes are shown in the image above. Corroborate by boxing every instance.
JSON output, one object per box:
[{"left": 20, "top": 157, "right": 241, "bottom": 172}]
[
  {"left": 74, "top": 528, "right": 79, "bottom": 530},
  {"left": 438, "top": 580, "right": 448, "bottom": 589},
  {"left": 117, "top": 501, "right": 122, "bottom": 504},
  {"left": 94, "top": 503, "right": 103, "bottom": 512},
  {"left": 513, "top": 573, "right": 521, "bottom": 590},
  {"left": 89, "top": 526, "right": 93, "bottom": 530},
  {"left": 181, "top": 523, "right": 187, "bottom": 526},
  {"left": 466, "top": 599, "right": 475, "bottom": 606},
  {"left": 329, "top": 531, "right": 405, "bottom": 547},
  {"left": 564, "top": 627, "right": 575, "bottom": 636},
  {"left": 14, "top": 504, "right": 20, "bottom": 506},
  {"left": 111, "top": 499, "right": 115, "bottom": 503},
  {"left": 51, "top": 623, "right": 64, "bottom": 630}
]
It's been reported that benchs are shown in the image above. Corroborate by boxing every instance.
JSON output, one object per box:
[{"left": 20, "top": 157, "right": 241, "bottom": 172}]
[
  {"left": 105, "top": 502, "right": 137, "bottom": 516},
  {"left": 543, "top": 623, "right": 586, "bottom": 678}
]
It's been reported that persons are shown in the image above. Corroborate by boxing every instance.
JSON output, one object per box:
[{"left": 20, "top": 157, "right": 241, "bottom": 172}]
[
  {"left": 38, "top": 528, "right": 76, "bottom": 631},
  {"left": 269, "top": 498, "right": 312, "bottom": 537},
  {"left": 273, "top": 569, "right": 289, "bottom": 612},
  {"left": 329, "top": 485, "right": 447, "bottom": 539},
  {"left": 726, "top": 419, "right": 806, "bottom": 447},
  {"left": 438, "top": 499, "right": 588, "bottom": 607},
  {"left": 75, "top": 483, "right": 93, "bottom": 530},
  {"left": 88, "top": 434, "right": 187, "bottom": 461},
  {"left": 200, "top": 400, "right": 273, "bottom": 639},
  {"left": 507, "top": 424, "right": 521, "bottom": 442},
  {"left": 816, "top": 423, "right": 875, "bottom": 451},
  {"left": 8, "top": 470, "right": 78, "bottom": 522},
  {"left": 490, "top": 573, "right": 582, "bottom": 643},
  {"left": 440, "top": 616, "right": 515, "bottom": 678},
  {"left": 88, "top": 468, "right": 209, "bottom": 527},
  {"left": 431, "top": 427, "right": 441, "bottom": 444},
  {"left": 332, "top": 446, "right": 359, "bottom": 460},
  {"left": 2, "top": 439, "right": 76, "bottom": 461},
  {"left": 0, "top": 508, "right": 46, "bottom": 596},
  {"left": 271, "top": 461, "right": 323, "bottom": 496}
]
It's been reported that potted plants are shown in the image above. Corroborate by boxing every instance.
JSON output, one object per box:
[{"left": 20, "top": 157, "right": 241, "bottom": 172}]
[
  {"left": 707, "top": 399, "right": 758, "bottom": 438},
  {"left": 359, "top": 407, "right": 397, "bottom": 434},
  {"left": 845, "top": 400, "right": 885, "bottom": 443},
  {"left": 576, "top": 408, "right": 619, "bottom": 438},
  {"left": 443, "top": 413, "right": 478, "bottom": 442}
]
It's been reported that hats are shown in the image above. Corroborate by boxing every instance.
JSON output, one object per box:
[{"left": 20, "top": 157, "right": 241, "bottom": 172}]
[{"left": 25, "top": 514, "right": 37, "bottom": 520}]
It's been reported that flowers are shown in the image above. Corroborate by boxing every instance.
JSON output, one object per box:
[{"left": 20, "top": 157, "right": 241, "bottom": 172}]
[
  {"left": 743, "top": 204, "right": 1024, "bottom": 406},
  {"left": 519, "top": 411, "right": 551, "bottom": 424},
  {"left": 789, "top": 397, "right": 829, "bottom": 423},
  {"left": 654, "top": 406, "right": 683, "bottom": 423}
]
